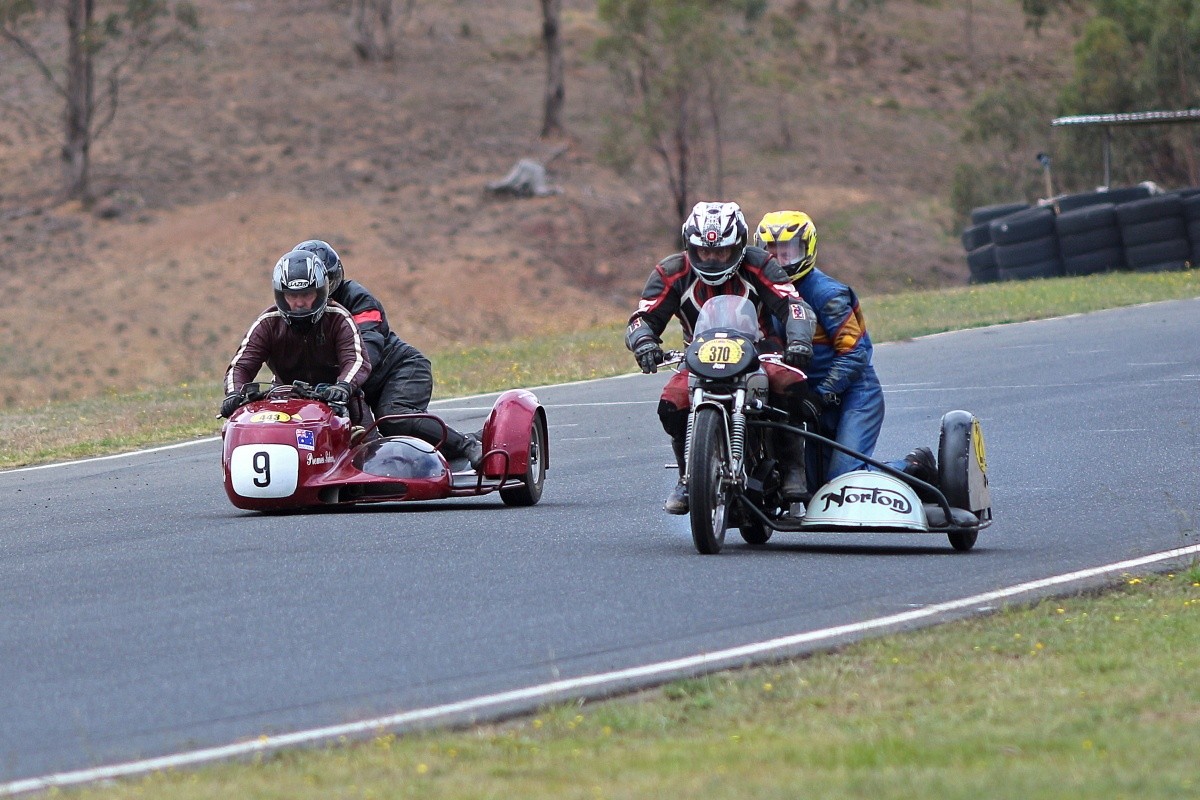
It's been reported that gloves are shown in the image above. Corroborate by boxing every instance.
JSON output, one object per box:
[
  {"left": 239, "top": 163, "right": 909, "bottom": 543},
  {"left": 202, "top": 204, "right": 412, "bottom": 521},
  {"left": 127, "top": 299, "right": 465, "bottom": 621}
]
[
  {"left": 324, "top": 384, "right": 349, "bottom": 403},
  {"left": 633, "top": 342, "right": 664, "bottom": 375},
  {"left": 801, "top": 392, "right": 823, "bottom": 419},
  {"left": 786, "top": 344, "right": 814, "bottom": 369},
  {"left": 220, "top": 396, "right": 238, "bottom": 417}
]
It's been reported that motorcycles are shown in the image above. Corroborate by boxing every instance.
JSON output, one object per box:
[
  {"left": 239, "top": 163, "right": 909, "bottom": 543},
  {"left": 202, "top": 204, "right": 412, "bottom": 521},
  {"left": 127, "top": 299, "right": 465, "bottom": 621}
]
[
  {"left": 215, "top": 379, "right": 549, "bottom": 513},
  {"left": 641, "top": 294, "right": 994, "bottom": 555}
]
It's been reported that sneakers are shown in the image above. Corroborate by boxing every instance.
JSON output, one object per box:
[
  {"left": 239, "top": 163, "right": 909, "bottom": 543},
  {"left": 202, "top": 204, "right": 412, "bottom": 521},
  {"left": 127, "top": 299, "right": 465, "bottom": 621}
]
[{"left": 906, "top": 448, "right": 938, "bottom": 504}]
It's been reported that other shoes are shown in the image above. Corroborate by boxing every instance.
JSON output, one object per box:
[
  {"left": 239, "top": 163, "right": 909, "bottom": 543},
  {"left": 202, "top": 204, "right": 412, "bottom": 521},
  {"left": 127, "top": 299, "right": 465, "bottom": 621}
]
[{"left": 464, "top": 431, "right": 485, "bottom": 468}]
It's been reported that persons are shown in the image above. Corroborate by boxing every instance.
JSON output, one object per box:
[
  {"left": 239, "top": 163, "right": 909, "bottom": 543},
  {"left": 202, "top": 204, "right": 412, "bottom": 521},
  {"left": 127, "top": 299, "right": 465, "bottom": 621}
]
[
  {"left": 294, "top": 240, "right": 483, "bottom": 470},
  {"left": 753, "top": 210, "right": 937, "bottom": 496},
  {"left": 625, "top": 202, "right": 817, "bottom": 516},
  {"left": 219, "top": 250, "right": 386, "bottom": 455}
]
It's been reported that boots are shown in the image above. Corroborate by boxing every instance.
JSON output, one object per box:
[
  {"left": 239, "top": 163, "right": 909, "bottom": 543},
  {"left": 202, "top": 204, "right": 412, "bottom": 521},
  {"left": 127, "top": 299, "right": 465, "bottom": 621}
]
[
  {"left": 779, "top": 426, "right": 808, "bottom": 500},
  {"left": 663, "top": 441, "right": 689, "bottom": 514}
]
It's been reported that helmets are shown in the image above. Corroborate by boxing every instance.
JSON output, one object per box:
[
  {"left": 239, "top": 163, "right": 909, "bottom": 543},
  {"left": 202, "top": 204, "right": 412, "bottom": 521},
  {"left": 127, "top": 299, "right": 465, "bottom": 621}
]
[
  {"left": 753, "top": 212, "right": 820, "bottom": 282},
  {"left": 681, "top": 199, "right": 748, "bottom": 287},
  {"left": 273, "top": 249, "right": 329, "bottom": 326},
  {"left": 294, "top": 241, "right": 343, "bottom": 295}
]
